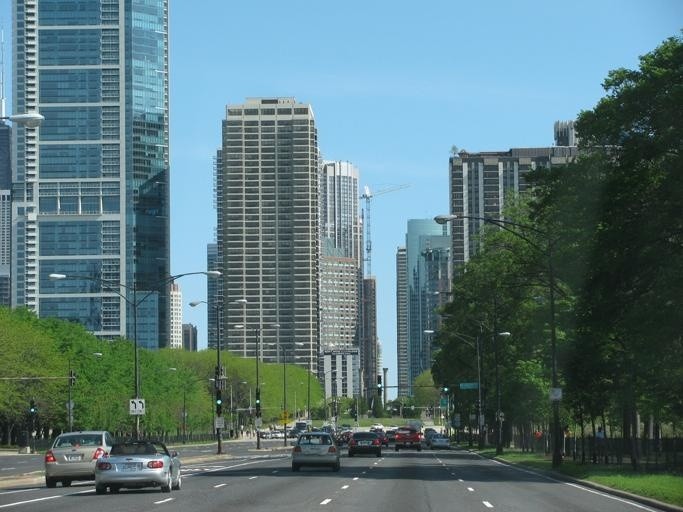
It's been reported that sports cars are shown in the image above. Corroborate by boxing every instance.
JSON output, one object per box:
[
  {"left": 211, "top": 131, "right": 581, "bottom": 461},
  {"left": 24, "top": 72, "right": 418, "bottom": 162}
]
[{"left": 93, "top": 440, "right": 183, "bottom": 493}]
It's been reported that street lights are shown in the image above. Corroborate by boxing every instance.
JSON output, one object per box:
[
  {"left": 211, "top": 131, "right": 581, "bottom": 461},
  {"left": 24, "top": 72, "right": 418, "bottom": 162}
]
[
  {"left": 187, "top": 298, "right": 248, "bottom": 455},
  {"left": 220, "top": 321, "right": 346, "bottom": 449},
  {"left": 140, "top": 367, "right": 176, "bottom": 377},
  {"left": 67, "top": 352, "right": 102, "bottom": 432},
  {"left": 48, "top": 269, "right": 222, "bottom": 439},
  {"left": 0, "top": 111, "right": 45, "bottom": 131},
  {"left": 216, "top": 390, "right": 222, "bottom": 414},
  {"left": 182, "top": 377, "right": 215, "bottom": 442},
  {"left": 421, "top": 329, "right": 512, "bottom": 450},
  {"left": 434, "top": 213, "right": 565, "bottom": 470},
  {"left": 433, "top": 291, "right": 451, "bottom": 295}
]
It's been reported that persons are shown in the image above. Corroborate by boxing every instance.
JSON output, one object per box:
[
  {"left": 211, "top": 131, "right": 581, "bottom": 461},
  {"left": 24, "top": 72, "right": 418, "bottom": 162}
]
[
  {"left": 563, "top": 425, "right": 573, "bottom": 437},
  {"left": 595, "top": 425, "right": 606, "bottom": 439},
  {"left": 533, "top": 428, "right": 543, "bottom": 438}
]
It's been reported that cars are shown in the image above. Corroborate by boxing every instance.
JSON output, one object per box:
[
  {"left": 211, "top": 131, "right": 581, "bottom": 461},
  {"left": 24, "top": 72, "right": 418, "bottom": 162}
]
[
  {"left": 45, "top": 431, "right": 112, "bottom": 487},
  {"left": 291, "top": 432, "right": 339, "bottom": 471},
  {"left": 258, "top": 419, "right": 396, "bottom": 445},
  {"left": 347, "top": 432, "right": 381, "bottom": 457},
  {"left": 424, "top": 427, "right": 450, "bottom": 450}
]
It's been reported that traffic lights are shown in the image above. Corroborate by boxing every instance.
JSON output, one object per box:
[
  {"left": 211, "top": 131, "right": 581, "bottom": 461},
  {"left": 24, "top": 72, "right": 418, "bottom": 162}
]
[
  {"left": 377, "top": 375, "right": 382, "bottom": 394},
  {"left": 29, "top": 400, "right": 35, "bottom": 416},
  {"left": 441, "top": 386, "right": 450, "bottom": 395}
]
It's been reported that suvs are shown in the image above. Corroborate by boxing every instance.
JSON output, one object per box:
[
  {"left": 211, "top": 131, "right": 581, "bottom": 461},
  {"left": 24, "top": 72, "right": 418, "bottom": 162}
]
[{"left": 394, "top": 426, "right": 423, "bottom": 452}]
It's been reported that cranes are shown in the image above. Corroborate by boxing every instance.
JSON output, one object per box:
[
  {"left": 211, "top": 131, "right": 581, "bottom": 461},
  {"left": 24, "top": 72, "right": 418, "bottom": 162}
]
[{"left": 361, "top": 182, "right": 414, "bottom": 398}]
